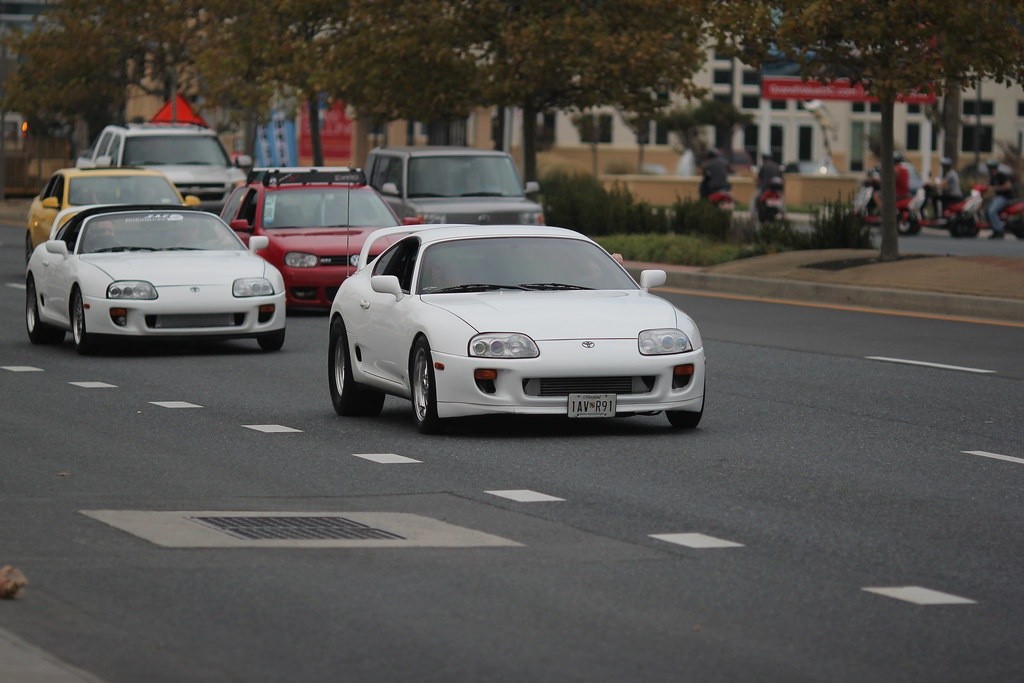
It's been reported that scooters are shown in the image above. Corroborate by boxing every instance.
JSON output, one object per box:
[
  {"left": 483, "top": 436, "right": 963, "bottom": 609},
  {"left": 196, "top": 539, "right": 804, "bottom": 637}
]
[
  {"left": 841, "top": 164, "right": 926, "bottom": 236},
  {"left": 948, "top": 181, "right": 1023, "bottom": 242},
  {"left": 895, "top": 172, "right": 983, "bottom": 238},
  {"left": 751, "top": 164, "right": 790, "bottom": 235},
  {"left": 708, "top": 182, "right": 736, "bottom": 227}
]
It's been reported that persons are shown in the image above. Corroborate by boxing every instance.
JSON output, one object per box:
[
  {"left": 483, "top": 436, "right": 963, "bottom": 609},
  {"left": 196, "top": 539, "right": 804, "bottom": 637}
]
[
  {"left": 87, "top": 220, "right": 123, "bottom": 250},
  {"left": 893, "top": 155, "right": 922, "bottom": 199},
  {"left": 754, "top": 151, "right": 784, "bottom": 208},
  {"left": 75, "top": 184, "right": 99, "bottom": 204},
  {"left": 466, "top": 170, "right": 482, "bottom": 195},
  {"left": 873, "top": 151, "right": 910, "bottom": 224},
  {"left": 388, "top": 158, "right": 413, "bottom": 193},
  {"left": 425, "top": 251, "right": 454, "bottom": 289},
  {"left": 173, "top": 220, "right": 235, "bottom": 249},
  {"left": 926, "top": 157, "right": 963, "bottom": 222},
  {"left": 700, "top": 147, "right": 734, "bottom": 197},
  {"left": 982, "top": 160, "right": 1014, "bottom": 240},
  {"left": 264, "top": 201, "right": 297, "bottom": 229},
  {"left": 135, "top": 182, "right": 161, "bottom": 204}
]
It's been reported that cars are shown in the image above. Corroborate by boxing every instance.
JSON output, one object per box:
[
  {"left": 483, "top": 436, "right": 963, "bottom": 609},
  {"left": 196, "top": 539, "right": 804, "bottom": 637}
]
[
  {"left": 24, "top": 166, "right": 203, "bottom": 263},
  {"left": 215, "top": 166, "right": 425, "bottom": 316}
]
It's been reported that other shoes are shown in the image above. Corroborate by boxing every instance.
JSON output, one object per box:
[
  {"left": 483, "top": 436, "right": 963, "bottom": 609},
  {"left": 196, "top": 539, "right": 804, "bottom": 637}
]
[{"left": 991, "top": 232, "right": 1004, "bottom": 239}]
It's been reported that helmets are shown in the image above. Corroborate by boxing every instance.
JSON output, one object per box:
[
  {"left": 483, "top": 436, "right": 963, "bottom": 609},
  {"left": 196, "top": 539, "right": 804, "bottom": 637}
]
[{"left": 985, "top": 160, "right": 998, "bottom": 167}]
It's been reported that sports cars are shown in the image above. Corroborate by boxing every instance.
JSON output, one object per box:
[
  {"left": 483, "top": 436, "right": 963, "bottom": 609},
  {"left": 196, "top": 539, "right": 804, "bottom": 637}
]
[
  {"left": 23, "top": 203, "right": 289, "bottom": 354},
  {"left": 327, "top": 224, "right": 707, "bottom": 430}
]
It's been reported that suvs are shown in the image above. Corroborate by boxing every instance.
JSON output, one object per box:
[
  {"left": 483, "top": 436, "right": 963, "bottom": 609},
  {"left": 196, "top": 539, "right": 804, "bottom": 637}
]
[
  {"left": 361, "top": 145, "right": 547, "bottom": 241},
  {"left": 86, "top": 122, "right": 259, "bottom": 215}
]
